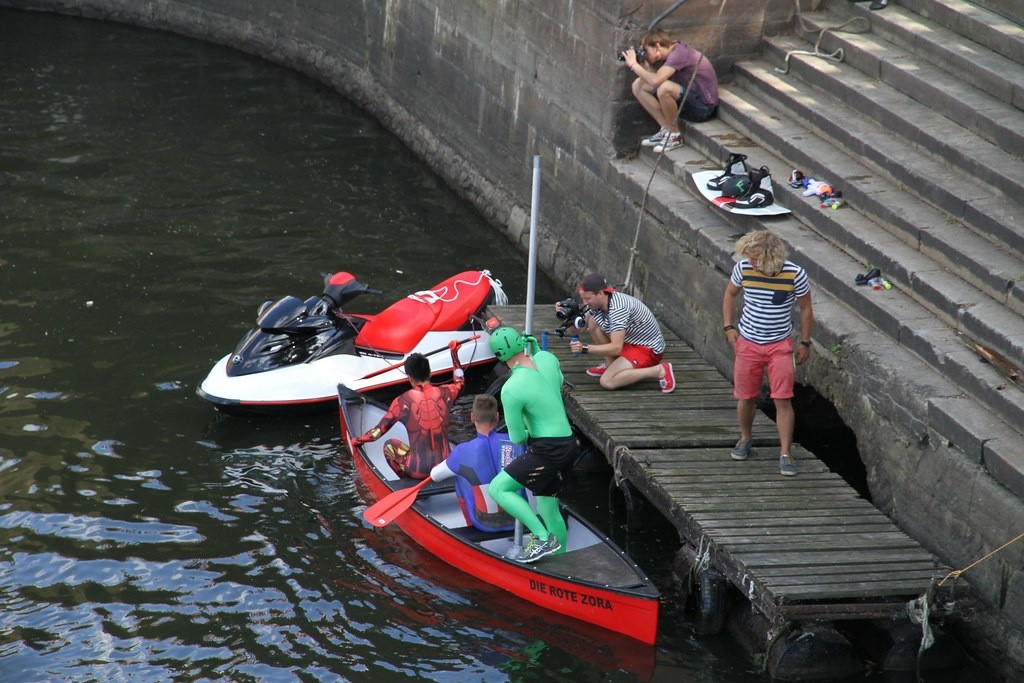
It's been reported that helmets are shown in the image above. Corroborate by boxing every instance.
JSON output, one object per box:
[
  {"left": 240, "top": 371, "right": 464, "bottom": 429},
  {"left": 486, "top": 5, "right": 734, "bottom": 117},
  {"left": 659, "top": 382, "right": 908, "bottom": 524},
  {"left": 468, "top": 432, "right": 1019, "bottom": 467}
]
[{"left": 490, "top": 327, "right": 524, "bottom": 362}]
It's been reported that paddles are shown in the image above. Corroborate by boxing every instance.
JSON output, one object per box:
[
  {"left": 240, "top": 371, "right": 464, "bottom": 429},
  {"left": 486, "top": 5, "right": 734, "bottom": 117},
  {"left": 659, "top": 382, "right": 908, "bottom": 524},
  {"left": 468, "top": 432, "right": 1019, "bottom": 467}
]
[
  {"left": 363, "top": 424, "right": 506, "bottom": 527},
  {"left": 359, "top": 334, "right": 483, "bottom": 382}
]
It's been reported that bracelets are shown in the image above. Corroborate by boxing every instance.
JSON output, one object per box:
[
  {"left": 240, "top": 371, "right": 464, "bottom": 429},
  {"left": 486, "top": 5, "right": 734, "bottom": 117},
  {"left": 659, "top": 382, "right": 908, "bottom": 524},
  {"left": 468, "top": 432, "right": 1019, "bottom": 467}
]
[
  {"left": 723, "top": 325, "right": 736, "bottom": 332},
  {"left": 628, "top": 62, "right": 638, "bottom": 71},
  {"left": 801, "top": 340, "right": 812, "bottom": 346}
]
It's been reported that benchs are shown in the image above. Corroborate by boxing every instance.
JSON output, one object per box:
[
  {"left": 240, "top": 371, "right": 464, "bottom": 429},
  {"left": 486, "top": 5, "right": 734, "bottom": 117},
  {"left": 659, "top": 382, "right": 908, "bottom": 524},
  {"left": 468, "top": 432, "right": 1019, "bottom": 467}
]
[
  {"left": 450, "top": 513, "right": 568, "bottom": 544},
  {"left": 388, "top": 473, "right": 455, "bottom": 500}
]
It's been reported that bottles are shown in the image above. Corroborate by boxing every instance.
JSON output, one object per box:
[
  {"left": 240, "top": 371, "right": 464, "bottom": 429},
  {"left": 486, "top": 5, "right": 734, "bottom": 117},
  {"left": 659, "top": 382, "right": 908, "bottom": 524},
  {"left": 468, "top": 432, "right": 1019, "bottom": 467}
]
[
  {"left": 572, "top": 334, "right": 578, "bottom": 357},
  {"left": 868, "top": 276, "right": 881, "bottom": 290},
  {"left": 821, "top": 197, "right": 845, "bottom": 210},
  {"left": 880, "top": 277, "right": 891, "bottom": 289}
]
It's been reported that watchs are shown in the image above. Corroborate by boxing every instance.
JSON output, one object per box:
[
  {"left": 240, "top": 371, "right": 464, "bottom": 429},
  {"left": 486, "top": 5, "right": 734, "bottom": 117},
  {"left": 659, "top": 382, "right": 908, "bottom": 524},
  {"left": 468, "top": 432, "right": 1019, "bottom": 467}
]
[{"left": 582, "top": 343, "right": 588, "bottom": 354}]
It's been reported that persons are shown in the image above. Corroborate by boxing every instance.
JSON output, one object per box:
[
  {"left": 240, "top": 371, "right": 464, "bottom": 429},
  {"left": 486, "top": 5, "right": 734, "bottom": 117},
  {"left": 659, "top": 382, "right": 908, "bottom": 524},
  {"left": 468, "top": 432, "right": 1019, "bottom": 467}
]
[
  {"left": 488, "top": 326, "right": 581, "bottom": 564},
  {"left": 555, "top": 273, "right": 675, "bottom": 393},
  {"left": 430, "top": 393, "right": 528, "bottom": 533},
  {"left": 621, "top": 29, "right": 718, "bottom": 153},
  {"left": 723, "top": 231, "right": 813, "bottom": 476},
  {"left": 351, "top": 339, "right": 465, "bottom": 479}
]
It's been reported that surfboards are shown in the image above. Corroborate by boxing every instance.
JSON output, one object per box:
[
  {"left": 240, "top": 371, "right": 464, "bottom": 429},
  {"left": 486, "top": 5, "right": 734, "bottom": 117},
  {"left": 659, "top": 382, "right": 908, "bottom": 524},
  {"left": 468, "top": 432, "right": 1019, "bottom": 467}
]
[{"left": 692, "top": 169, "right": 792, "bottom": 215}]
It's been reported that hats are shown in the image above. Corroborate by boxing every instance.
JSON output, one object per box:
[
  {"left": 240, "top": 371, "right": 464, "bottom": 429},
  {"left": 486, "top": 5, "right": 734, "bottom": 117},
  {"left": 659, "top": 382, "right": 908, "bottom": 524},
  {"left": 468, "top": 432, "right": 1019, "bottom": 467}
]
[
  {"left": 579, "top": 274, "right": 617, "bottom": 293},
  {"left": 722, "top": 175, "right": 752, "bottom": 197}
]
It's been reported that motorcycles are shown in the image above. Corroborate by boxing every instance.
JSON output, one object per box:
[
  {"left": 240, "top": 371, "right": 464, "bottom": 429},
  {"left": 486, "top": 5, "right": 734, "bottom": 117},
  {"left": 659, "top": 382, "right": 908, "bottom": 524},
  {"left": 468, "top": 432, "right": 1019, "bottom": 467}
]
[{"left": 196, "top": 269, "right": 499, "bottom": 407}]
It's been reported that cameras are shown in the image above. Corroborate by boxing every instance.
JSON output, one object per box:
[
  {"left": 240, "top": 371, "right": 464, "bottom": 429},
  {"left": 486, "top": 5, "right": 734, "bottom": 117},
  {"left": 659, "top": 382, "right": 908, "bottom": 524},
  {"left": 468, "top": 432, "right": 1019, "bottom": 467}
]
[
  {"left": 556, "top": 299, "right": 580, "bottom": 320},
  {"left": 618, "top": 46, "right": 646, "bottom": 63}
]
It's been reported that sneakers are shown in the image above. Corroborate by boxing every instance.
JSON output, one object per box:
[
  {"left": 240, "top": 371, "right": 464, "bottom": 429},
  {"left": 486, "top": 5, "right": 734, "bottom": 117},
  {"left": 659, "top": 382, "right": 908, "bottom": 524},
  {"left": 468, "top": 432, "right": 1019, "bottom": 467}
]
[
  {"left": 735, "top": 168, "right": 775, "bottom": 207},
  {"left": 642, "top": 127, "right": 685, "bottom": 152},
  {"left": 731, "top": 437, "right": 754, "bottom": 460},
  {"left": 780, "top": 454, "right": 798, "bottom": 476},
  {"left": 659, "top": 362, "right": 675, "bottom": 393},
  {"left": 515, "top": 533, "right": 562, "bottom": 564},
  {"left": 706, "top": 153, "right": 748, "bottom": 190},
  {"left": 586, "top": 363, "right": 607, "bottom": 377}
]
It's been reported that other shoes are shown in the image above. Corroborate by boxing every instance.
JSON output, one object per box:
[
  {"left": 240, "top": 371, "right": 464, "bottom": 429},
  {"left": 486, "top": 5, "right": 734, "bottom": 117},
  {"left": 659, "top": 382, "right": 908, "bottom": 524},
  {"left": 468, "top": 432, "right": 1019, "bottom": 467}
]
[{"left": 869, "top": 0, "right": 888, "bottom": 9}]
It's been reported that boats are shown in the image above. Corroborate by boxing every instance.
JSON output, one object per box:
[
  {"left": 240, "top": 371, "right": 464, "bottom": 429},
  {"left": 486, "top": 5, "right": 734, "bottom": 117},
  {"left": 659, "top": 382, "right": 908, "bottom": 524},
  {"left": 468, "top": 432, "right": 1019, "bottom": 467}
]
[{"left": 335, "top": 383, "right": 660, "bottom": 643}]
